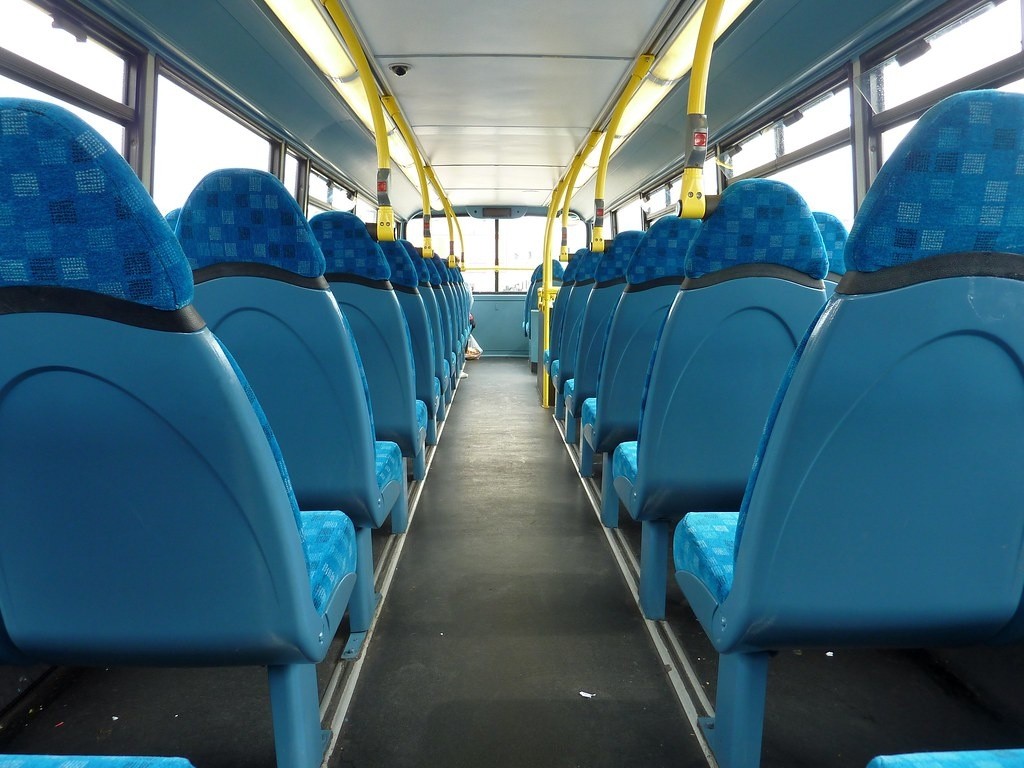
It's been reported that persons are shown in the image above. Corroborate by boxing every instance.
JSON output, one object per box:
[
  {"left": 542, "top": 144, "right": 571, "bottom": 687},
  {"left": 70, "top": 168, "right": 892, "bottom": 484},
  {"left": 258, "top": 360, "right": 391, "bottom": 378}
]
[{"left": 460, "top": 312, "right": 475, "bottom": 377}]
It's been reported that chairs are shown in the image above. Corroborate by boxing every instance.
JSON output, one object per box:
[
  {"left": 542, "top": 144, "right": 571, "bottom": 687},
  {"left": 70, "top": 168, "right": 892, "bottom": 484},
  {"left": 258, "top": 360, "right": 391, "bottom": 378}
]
[{"left": 0, "top": 89, "right": 1022, "bottom": 768}]
